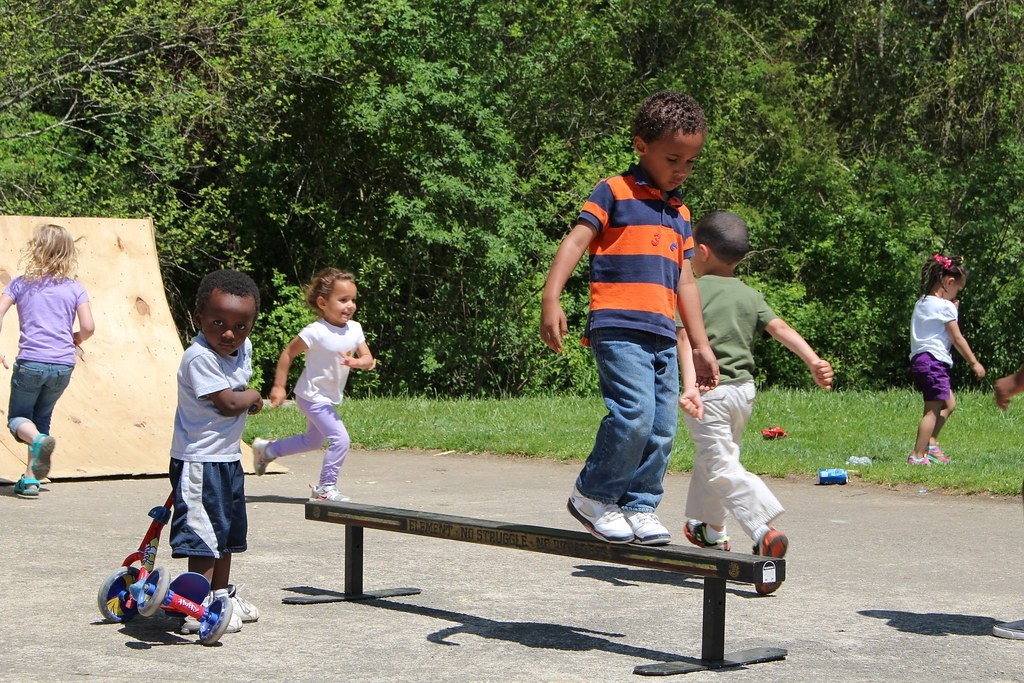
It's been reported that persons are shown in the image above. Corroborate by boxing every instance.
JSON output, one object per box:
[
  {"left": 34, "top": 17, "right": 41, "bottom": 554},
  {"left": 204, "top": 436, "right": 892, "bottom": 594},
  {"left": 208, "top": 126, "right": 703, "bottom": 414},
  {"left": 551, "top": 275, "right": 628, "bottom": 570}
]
[
  {"left": 540, "top": 90, "right": 719, "bottom": 544},
  {"left": 675, "top": 211, "right": 834, "bottom": 594},
  {"left": 992, "top": 366, "right": 1024, "bottom": 639},
  {"left": 252, "top": 267, "right": 373, "bottom": 503},
  {"left": 0, "top": 224, "right": 95, "bottom": 499},
  {"left": 907, "top": 255, "right": 985, "bottom": 465},
  {"left": 168, "top": 274, "right": 264, "bottom": 633}
]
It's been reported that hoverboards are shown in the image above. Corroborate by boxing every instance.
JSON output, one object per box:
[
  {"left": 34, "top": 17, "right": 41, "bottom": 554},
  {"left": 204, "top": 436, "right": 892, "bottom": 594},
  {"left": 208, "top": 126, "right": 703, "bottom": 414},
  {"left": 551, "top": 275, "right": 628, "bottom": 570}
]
[{"left": 98, "top": 405, "right": 259, "bottom": 646}]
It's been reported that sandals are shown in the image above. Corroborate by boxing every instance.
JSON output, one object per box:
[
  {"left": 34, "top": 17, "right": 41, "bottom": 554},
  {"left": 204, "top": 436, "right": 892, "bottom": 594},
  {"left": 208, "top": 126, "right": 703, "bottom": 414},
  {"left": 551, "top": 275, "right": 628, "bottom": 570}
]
[
  {"left": 29, "top": 434, "right": 56, "bottom": 480},
  {"left": 14, "top": 474, "right": 41, "bottom": 498}
]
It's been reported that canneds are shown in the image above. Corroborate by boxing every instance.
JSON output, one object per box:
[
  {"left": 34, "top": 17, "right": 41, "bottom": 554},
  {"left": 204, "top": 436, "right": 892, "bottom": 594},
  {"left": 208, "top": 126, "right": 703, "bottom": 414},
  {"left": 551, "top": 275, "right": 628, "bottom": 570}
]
[{"left": 818, "top": 467, "right": 848, "bottom": 485}]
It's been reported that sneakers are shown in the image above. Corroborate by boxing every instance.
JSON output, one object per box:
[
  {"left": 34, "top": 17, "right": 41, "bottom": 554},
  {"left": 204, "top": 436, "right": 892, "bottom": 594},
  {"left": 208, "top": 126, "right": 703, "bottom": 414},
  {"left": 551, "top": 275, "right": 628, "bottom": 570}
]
[
  {"left": 309, "top": 482, "right": 352, "bottom": 503},
  {"left": 924, "top": 445, "right": 951, "bottom": 466},
  {"left": 252, "top": 437, "right": 277, "bottom": 476},
  {"left": 567, "top": 484, "right": 636, "bottom": 545},
  {"left": 752, "top": 526, "right": 789, "bottom": 596},
  {"left": 908, "top": 454, "right": 933, "bottom": 467},
  {"left": 684, "top": 518, "right": 732, "bottom": 552},
  {"left": 213, "top": 584, "right": 260, "bottom": 623},
  {"left": 621, "top": 508, "right": 674, "bottom": 545},
  {"left": 180, "top": 590, "right": 243, "bottom": 635}
]
